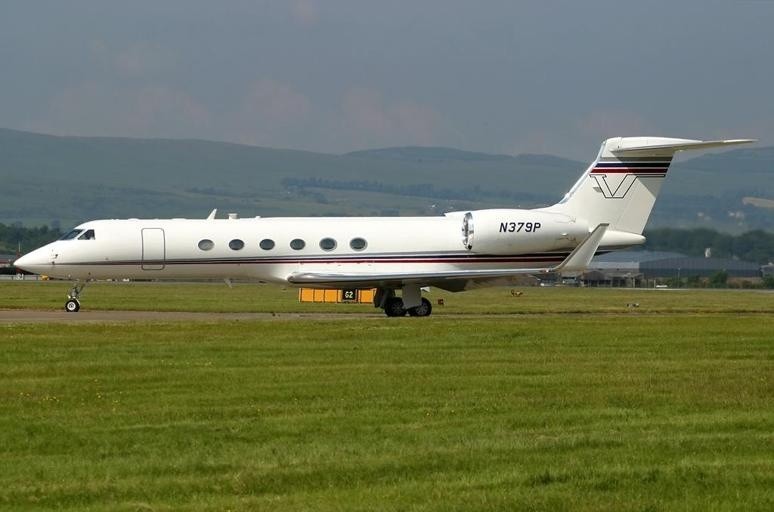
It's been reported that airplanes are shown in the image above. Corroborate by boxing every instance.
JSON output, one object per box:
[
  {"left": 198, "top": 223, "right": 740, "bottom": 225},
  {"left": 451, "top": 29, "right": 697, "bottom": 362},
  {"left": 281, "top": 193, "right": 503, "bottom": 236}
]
[{"left": 13, "top": 136, "right": 759, "bottom": 318}]
